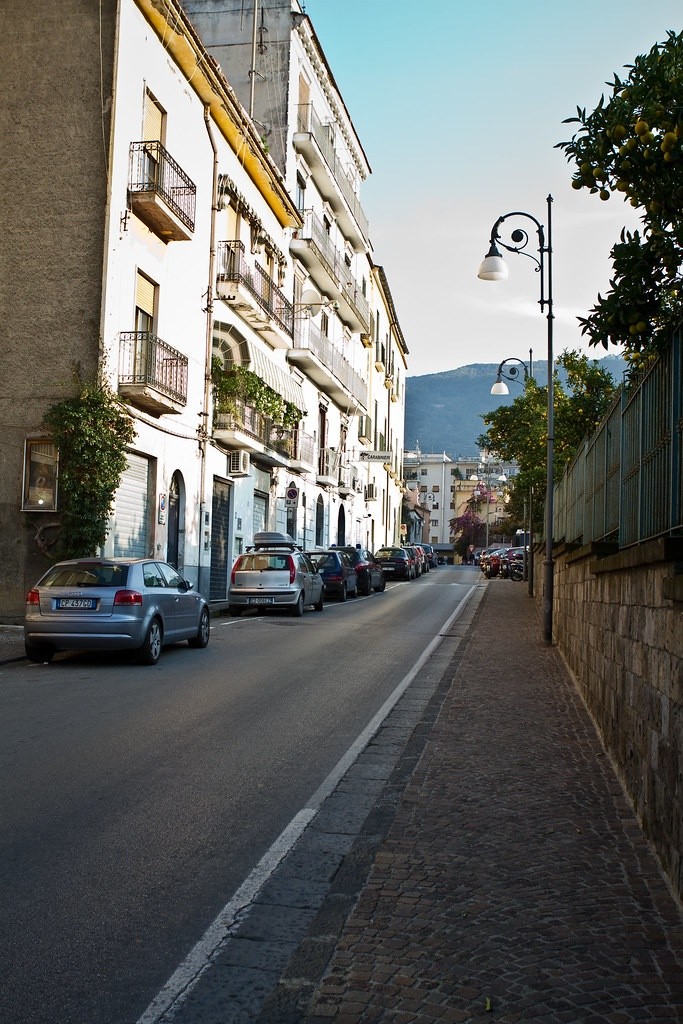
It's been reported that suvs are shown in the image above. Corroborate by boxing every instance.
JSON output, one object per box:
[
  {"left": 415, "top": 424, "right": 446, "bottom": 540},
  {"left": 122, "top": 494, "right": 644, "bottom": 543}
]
[
  {"left": 329, "top": 544, "right": 386, "bottom": 597},
  {"left": 226, "top": 531, "right": 326, "bottom": 618}
]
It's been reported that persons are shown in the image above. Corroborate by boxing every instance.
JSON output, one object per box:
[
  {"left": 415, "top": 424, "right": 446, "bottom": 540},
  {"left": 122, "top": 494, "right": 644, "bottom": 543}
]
[
  {"left": 462, "top": 554, "right": 468, "bottom": 565},
  {"left": 469, "top": 552, "right": 474, "bottom": 564}
]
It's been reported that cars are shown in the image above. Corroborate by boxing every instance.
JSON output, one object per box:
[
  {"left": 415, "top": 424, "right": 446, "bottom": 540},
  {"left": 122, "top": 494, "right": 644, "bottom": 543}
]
[
  {"left": 24, "top": 556, "right": 212, "bottom": 665},
  {"left": 373, "top": 543, "right": 439, "bottom": 582},
  {"left": 299, "top": 549, "right": 359, "bottom": 602},
  {"left": 474, "top": 545, "right": 528, "bottom": 583}
]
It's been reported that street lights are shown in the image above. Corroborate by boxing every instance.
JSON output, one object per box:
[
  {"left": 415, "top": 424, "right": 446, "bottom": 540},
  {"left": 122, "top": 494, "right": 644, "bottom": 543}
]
[
  {"left": 473, "top": 193, "right": 558, "bottom": 652},
  {"left": 421, "top": 491, "right": 437, "bottom": 546},
  {"left": 488, "top": 347, "right": 536, "bottom": 599},
  {"left": 469, "top": 457, "right": 507, "bottom": 550}
]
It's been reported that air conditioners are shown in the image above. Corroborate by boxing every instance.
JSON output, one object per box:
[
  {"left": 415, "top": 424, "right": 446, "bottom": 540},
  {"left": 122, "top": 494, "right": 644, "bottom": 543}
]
[
  {"left": 231, "top": 449, "right": 250, "bottom": 474},
  {"left": 367, "top": 483, "right": 377, "bottom": 501}
]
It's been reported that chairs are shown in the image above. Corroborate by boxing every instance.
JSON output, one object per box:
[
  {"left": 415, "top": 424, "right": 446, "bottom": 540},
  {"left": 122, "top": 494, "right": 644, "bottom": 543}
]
[{"left": 112, "top": 572, "right": 128, "bottom": 586}]
